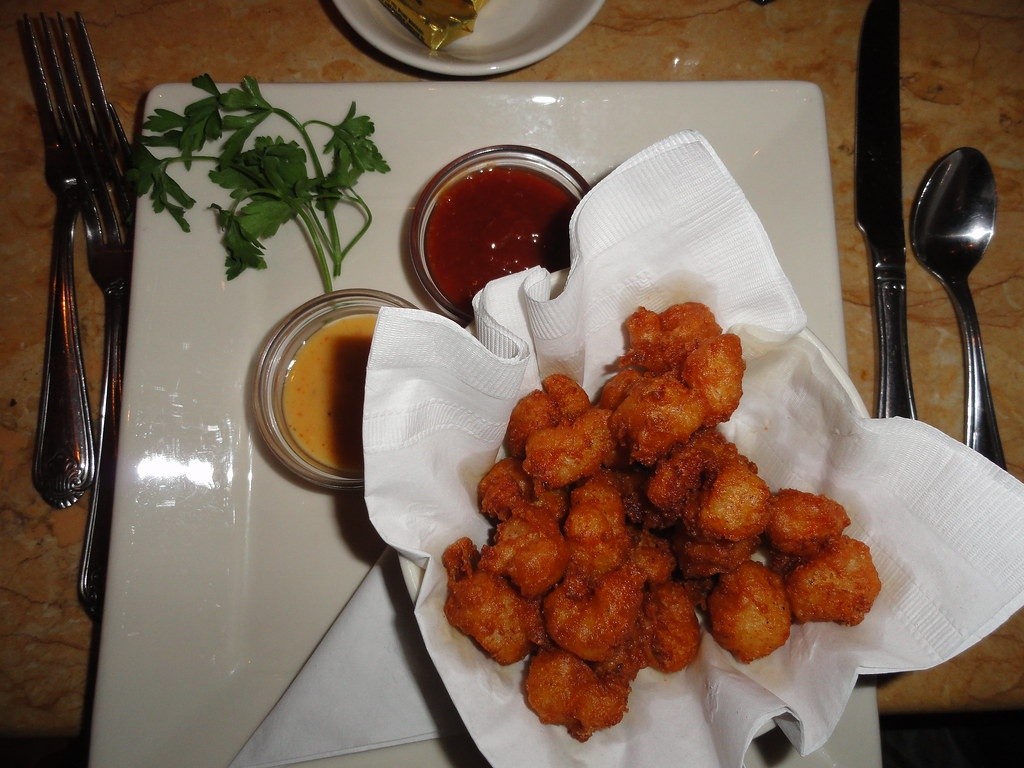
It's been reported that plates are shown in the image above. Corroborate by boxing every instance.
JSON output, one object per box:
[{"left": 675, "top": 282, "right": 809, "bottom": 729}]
[
  {"left": 76, "top": 75, "right": 890, "bottom": 768},
  {"left": 329, "top": 0, "right": 605, "bottom": 77}
]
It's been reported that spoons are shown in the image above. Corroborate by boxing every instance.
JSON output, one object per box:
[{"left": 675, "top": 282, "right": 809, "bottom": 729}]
[{"left": 910, "top": 146, "right": 1007, "bottom": 472}]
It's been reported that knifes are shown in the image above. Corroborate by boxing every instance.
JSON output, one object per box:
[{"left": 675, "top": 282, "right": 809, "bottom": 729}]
[{"left": 853, "top": 0, "right": 918, "bottom": 423}]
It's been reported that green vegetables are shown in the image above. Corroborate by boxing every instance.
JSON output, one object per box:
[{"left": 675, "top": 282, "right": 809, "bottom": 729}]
[{"left": 124, "top": 70, "right": 392, "bottom": 301}]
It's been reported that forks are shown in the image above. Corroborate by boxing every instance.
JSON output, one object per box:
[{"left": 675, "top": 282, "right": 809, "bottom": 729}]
[
  {"left": 16, "top": 12, "right": 112, "bottom": 512},
  {"left": 44, "top": 102, "right": 141, "bottom": 623}
]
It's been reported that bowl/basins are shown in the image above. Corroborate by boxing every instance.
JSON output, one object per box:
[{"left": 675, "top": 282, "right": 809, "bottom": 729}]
[
  {"left": 251, "top": 287, "right": 428, "bottom": 491},
  {"left": 407, "top": 143, "right": 593, "bottom": 326}
]
